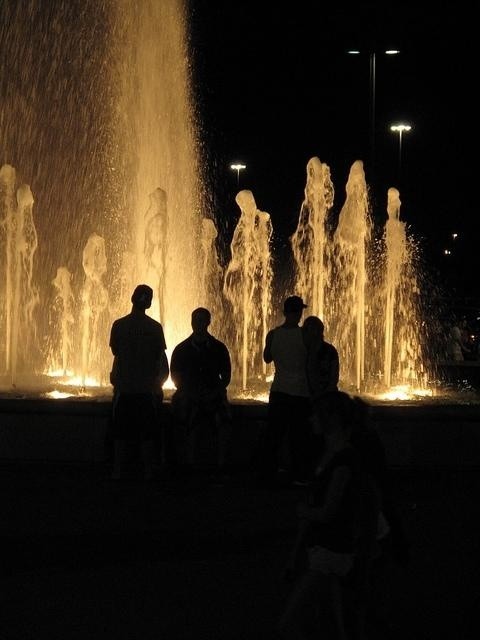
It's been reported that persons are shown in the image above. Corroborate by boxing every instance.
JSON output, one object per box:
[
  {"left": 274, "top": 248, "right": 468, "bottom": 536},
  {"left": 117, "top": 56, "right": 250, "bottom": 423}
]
[
  {"left": 107, "top": 283, "right": 171, "bottom": 482},
  {"left": 267, "top": 389, "right": 380, "bottom": 638},
  {"left": 446, "top": 317, "right": 472, "bottom": 362},
  {"left": 263, "top": 295, "right": 321, "bottom": 491},
  {"left": 301, "top": 315, "right": 339, "bottom": 405},
  {"left": 165, "top": 303, "right": 233, "bottom": 491}
]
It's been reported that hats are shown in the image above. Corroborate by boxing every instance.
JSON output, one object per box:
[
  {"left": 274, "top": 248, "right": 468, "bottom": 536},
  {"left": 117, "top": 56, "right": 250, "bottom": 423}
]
[{"left": 283, "top": 296, "right": 308, "bottom": 309}]
